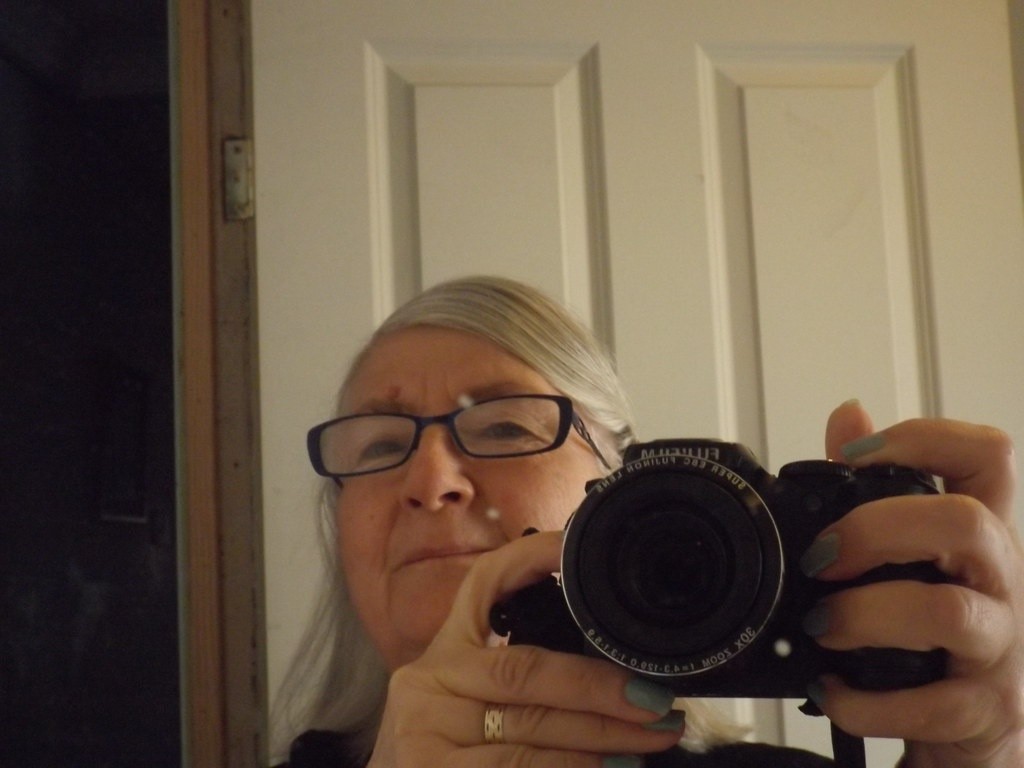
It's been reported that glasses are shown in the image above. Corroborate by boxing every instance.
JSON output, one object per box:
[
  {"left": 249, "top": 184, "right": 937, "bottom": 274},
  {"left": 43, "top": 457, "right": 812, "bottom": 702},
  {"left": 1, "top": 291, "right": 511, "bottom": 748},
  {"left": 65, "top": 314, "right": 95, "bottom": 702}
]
[{"left": 307, "top": 394, "right": 612, "bottom": 489}]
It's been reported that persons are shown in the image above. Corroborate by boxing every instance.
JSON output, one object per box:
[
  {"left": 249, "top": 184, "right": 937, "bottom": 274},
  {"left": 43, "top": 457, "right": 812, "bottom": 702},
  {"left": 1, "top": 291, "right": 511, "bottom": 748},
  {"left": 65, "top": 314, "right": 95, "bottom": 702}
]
[{"left": 264, "top": 274, "right": 1024, "bottom": 768}]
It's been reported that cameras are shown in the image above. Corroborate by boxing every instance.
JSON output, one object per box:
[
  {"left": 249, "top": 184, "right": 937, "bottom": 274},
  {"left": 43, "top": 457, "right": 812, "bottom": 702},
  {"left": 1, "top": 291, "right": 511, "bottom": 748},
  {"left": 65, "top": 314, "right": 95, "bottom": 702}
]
[{"left": 508, "top": 432, "right": 954, "bottom": 699}]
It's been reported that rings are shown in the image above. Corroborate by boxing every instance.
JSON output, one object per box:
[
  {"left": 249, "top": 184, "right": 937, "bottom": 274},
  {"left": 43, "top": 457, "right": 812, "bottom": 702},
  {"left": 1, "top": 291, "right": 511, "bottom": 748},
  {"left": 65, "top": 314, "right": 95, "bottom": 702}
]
[{"left": 484, "top": 703, "right": 506, "bottom": 744}]
